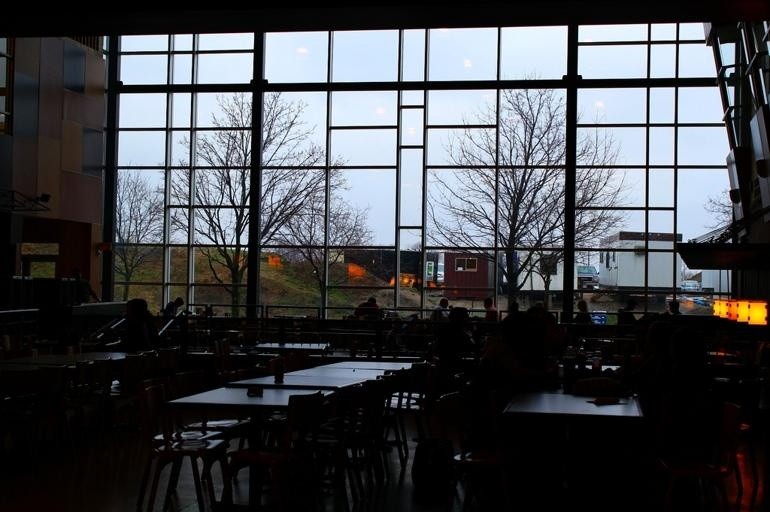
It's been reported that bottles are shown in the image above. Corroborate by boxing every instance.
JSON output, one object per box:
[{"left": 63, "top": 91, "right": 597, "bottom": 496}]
[
  {"left": 592, "top": 350, "right": 603, "bottom": 372},
  {"left": 563, "top": 346, "right": 575, "bottom": 377},
  {"left": 577, "top": 347, "right": 586, "bottom": 370}
]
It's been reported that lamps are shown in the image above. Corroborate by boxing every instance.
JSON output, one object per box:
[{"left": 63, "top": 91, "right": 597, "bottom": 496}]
[{"left": 712, "top": 300, "right": 768, "bottom": 325}]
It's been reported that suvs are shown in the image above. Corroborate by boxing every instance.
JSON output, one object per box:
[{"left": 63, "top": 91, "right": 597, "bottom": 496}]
[
  {"left": 437, "top": 263, "right": 444, "bottom": 282},
  {"left": 576, "top": 265, "right": 600, "bottom": 291}
]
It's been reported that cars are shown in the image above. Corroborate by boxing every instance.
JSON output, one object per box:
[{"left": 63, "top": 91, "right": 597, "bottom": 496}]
[{"left": 680, "top": 280, "right": 701, "bottom": 292}]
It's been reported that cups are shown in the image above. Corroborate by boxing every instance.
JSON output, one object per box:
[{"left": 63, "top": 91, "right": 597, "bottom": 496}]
[{"left": 272, "top": 358, "right": 286, "bottom": 385}]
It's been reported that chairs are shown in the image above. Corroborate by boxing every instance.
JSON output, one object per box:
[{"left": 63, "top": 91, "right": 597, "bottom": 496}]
[{"left": 1, "top": 312, "right": 770, "bottom": 510}]
